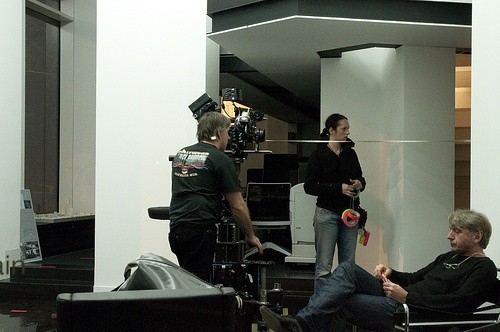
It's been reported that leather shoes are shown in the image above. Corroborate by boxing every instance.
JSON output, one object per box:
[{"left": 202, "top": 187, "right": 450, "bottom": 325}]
[{"left": 259, "top": 305, "right": 303, "bottom": 332}]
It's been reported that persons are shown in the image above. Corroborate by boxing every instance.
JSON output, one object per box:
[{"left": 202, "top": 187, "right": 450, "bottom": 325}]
[
  {"left": 168, "top": 112, "right": 263, "bottom": 283},
  {"left": 304, "top": 113, "right": 366, "bottom": 304},
  {"left": 260, "top": 208, "right": 497, "bottom": 332}
]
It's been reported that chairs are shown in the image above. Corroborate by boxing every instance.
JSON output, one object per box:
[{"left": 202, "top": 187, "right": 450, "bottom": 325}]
[
  {"left": 395, "top": 303, "right": 500, "bottom": 332},
  {"left": 244, "top": 183, "right": 292, "bottom": 258},
  {"left": 56, "top": 287, "right": 237, "bottom": 332}
]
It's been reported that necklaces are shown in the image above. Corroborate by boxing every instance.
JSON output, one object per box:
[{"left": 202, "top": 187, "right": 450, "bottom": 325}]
[{"left": 328, "top": 145, "right": 341, "bottom": 151}]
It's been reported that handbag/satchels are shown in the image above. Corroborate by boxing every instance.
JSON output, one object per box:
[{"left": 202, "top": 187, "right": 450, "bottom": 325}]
[
  {"left": 110, "top": 253, "right": 220, "bottom": 291},
  {"left": 356, "top": 206, "right": 367, "bottom": 229}
]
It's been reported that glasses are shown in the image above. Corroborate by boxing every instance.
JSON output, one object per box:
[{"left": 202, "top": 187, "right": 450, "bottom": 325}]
[{"left": 442, "top": 254, "right": 472, "bottom": 270}]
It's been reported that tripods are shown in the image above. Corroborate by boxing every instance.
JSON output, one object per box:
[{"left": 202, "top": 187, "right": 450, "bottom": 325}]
[{"left": 381, "top": 273, "right": 387, "bottom": 282}]
